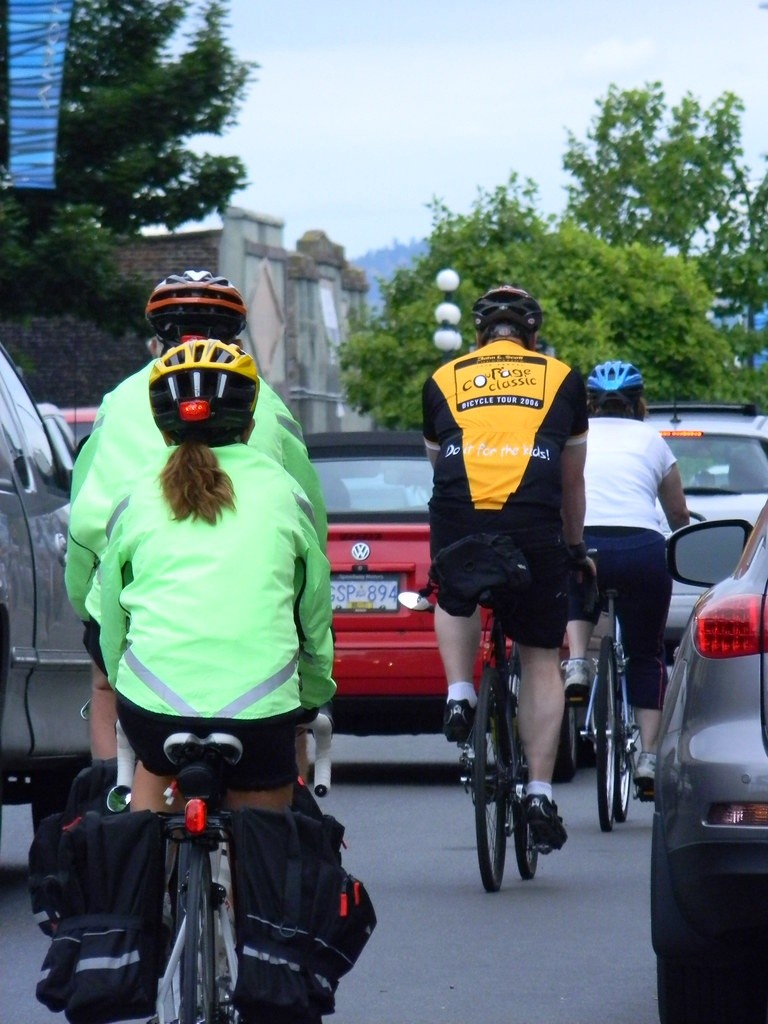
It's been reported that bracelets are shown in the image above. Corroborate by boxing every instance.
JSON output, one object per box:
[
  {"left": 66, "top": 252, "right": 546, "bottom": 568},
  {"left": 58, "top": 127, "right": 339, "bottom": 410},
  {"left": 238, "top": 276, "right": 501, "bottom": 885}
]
[{"left": 567, "top": 544, "right": 588, "bottom": 561}]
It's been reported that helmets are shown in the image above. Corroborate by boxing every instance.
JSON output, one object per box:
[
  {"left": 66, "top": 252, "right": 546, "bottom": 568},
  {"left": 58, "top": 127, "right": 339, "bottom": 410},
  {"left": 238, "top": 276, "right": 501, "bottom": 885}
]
[
  {"left": 148, "top": 338, "right": 260, "bottom": 447},
  {"left": 585, "top": 360, "right": 644, "bottom": 406},
  {"left": 473, "top": 285, "right": 543, "bottom": 331},
  {"left": 144, "top": 268, "right": 248, "bottom": 347}
]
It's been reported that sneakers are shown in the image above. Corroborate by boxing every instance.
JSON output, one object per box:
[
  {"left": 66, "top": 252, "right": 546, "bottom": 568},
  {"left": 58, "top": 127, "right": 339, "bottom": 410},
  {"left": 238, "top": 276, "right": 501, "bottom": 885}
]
[
  {"left": 565, "top": 659, "right": 595, "bottom": 699},
  {"left": 525, "top": 793, "right": 568, "bottom": 854},
  {"left": 632, "top": 752, "right": 656, "bottom": 790},
  {"left": 443, "top": 699, "right": 478, "bottom": 742}
]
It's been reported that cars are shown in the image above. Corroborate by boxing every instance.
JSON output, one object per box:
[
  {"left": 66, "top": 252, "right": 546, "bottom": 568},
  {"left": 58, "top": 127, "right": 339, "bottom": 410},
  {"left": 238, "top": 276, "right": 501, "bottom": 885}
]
[
  {"left": 652, "top": 494, "right": 767, "bottom": 1023},
  {"left": 0, "top": 347, "right": 94, "bottom": 804}
]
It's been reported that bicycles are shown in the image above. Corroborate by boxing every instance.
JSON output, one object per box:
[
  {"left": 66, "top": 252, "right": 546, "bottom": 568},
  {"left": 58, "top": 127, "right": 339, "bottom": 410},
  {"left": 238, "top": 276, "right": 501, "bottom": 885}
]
[
  {"left": 458, "top": 592, "right": 665, "bottom": 892},
  {"left": 115, "top": 712, "right": 330, "bottom": 1024}
]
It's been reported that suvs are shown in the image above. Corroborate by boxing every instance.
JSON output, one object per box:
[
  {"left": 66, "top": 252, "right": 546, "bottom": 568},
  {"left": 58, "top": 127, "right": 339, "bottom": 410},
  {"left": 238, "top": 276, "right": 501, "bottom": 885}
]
[
  {"left": 298, "top": 430, "right": 595, "bottom": 786},
  {"left": 641, "top": 403, "right": 768, "bottom": 638}
]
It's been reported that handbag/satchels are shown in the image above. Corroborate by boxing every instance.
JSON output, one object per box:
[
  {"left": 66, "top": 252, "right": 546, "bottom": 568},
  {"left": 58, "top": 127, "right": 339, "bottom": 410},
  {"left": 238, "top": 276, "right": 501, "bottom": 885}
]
[
  {"left": 225, "top": 806, "right": 379, "bottom": 1023},
  {"left": 26, "top": 809, "right": 166, "bottom": 1024}
]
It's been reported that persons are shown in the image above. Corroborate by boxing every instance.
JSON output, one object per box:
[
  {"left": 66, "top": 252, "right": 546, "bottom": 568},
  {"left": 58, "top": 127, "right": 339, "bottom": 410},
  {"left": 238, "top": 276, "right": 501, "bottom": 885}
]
[
  {"left": 420, "top": 287, "right": 600, "bottom": 849},
  {"left": 96, "top": 339, "right": 337, "bottom": 921},
  {"left": 64, "top": 271, "right": 329, "bottom": 785},
  {"left": 565, "top": 361, "right": 690, "bottom": 801}
]
